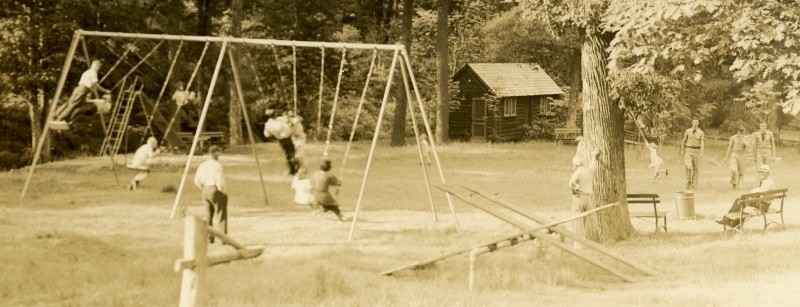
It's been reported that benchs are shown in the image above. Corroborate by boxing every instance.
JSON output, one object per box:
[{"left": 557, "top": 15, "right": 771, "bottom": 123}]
[
  {"left": 626, "top": 194, "right": 667, "bottom": 234},
  {"left": 724, "top": 188, "right": 789, "bottom": 234},
  {"left": 554, "top": 128, "right": 582, "bottom": 146},
  {"left": 176, "top": 131, "right": 226, "bottom": 153}
]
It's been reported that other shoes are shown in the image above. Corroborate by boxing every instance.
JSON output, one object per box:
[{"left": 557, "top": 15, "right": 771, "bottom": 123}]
[
  {"left": 715, "top": 220, "right": 724, "bottom": 224},
  {"left": 686, "top": 182, "right": 691, "bottom": 188},
  {"left": 129, "top": 185, "right": 132, "bottom": 192},
  {"left": 135, "top": 180, "right": 139, "bottom": 188}
]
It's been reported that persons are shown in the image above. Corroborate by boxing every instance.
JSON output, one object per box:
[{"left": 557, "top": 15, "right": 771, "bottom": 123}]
[
  {"left": 725, "top": 125, "right": 751, "bottom": 192},
  {"left": 569, "top": 147, "right": 601, "bottom": 250},
  {"left": 55, "top": 59, "right": 344, "bottom": 252},
  {"left": 645, "top": 138, "right": 668, "bottom": 178},
  {"left": 680, "top": 117, "right": 705, "bottom": 191},
  {"left": 714, "top": 164, "right": 776, "bottom": 232},
  {"left": 748, "top": 122, "right": 776, "bottom": 179}
]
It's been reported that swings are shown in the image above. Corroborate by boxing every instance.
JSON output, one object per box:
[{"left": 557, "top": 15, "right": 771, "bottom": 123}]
[
  {"left": 243, "top": 42, "right": 307, "bottom": 174},
  {"left": 125, "top": 41, "right": 211, "bottom": 171},
  {"left": 292, "top": 45, "right": 330, "bottom": 208},
  {"left": 50, "top": 40, "right": 165, "bottom": 130},
  {"left": 315, "top": 48, "right": 382, "bottom": 214}
]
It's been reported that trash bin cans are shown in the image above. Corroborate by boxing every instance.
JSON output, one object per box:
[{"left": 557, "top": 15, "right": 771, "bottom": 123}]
[{"left": 674, "top": 191, "right": 696, "bottom": 220}]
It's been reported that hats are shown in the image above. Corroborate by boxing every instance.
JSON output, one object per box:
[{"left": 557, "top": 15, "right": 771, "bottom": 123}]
[{"left": 758, "top": 164, "right": 769, "bottom": 173}]
